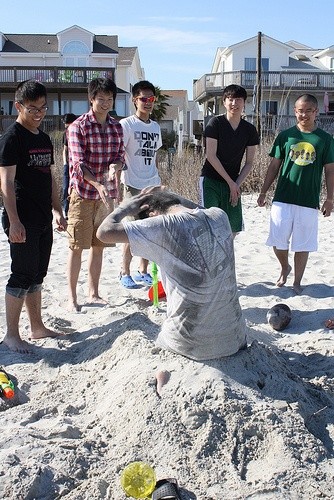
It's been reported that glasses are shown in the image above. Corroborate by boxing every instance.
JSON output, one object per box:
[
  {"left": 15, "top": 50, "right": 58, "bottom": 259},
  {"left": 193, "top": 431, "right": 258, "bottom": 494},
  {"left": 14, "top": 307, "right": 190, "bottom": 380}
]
[
  {"left": 296, "top": 109, "right": 318, "bottom": 114},
  {"left": 18, "top": 101, "right": 48, "bottom": 113},
  {"left": 135, "top": 96, "right": 157, "bottom": 102}
]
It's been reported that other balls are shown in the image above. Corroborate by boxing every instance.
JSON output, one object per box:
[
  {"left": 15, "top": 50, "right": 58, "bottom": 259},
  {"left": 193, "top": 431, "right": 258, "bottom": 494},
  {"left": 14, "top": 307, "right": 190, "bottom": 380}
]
[
  {"left": 267, "top": 304, "right": 292, "bottom": 331},
  {"left": 122, "top": 461, "right": 157, "bottom": 499}
]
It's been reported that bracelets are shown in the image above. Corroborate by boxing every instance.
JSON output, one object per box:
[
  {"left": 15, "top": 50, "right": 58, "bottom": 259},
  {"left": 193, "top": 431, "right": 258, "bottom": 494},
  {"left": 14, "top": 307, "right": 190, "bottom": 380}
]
[{"left": 259, "top": 192, "right": 267, "bottom": 195}]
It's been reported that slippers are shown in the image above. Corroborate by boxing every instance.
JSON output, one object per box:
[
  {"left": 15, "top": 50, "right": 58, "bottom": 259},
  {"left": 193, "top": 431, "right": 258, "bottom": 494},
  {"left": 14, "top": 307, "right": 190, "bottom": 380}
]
[
  {"left": 118, "top": 272, "right": 137, "bottom": 288},
  {"left": 134, "top": 274, "right": 154, "bottom": 285}
]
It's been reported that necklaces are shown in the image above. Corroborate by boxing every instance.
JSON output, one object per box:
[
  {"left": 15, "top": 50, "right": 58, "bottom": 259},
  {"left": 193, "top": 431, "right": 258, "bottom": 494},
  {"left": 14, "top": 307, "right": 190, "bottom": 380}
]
[{"left": 134, "top": 113, "right": 150, "bottom": 123}]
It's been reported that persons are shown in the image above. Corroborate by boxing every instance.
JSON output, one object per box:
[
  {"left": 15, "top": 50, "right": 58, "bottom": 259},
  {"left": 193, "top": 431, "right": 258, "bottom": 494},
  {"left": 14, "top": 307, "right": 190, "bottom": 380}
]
[
  {"left": 95, "top": 186, "right": 248, "bottom": 361},
  {"left": 61, "top": 113, "right": 76, "bottom": 222},
  {"left": 258, "top": 95, "right": 334, "bottom": 294},
  {"left": 67, "top": 77, "right": 125, "bottom": 313},
  {"left": 118, "top": 80, "right": 164, "bottom": 290},
  {"left": 0, "top": 78, "right": 67, "bottom": 354},
  {"left": 199, "top": 83, "right": 258, "bottom": 232}
]
